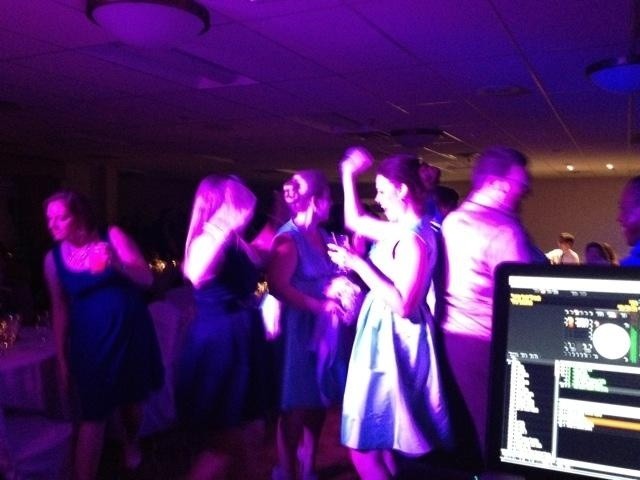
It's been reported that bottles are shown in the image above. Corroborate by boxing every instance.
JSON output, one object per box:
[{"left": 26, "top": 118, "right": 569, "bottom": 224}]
[{"left": 35, "top": 310, "right": 50, "bottom": 347}]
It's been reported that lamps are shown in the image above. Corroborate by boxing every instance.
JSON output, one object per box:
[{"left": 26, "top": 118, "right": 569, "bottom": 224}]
[{"left": 85, "top": 0, "right": 212, "bottom": 54}]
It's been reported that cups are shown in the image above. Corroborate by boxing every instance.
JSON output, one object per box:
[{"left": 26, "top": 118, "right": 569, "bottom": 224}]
[{"left": 331, "top": 234, "right": 350, "bottom": 274}]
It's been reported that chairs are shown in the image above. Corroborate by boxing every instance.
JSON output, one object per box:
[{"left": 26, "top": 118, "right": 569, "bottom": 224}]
[{"left": 1, "top": 284, "right": 197, "bottom": 480}]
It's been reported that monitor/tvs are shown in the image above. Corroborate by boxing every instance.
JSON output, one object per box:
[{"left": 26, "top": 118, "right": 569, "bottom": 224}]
[{"left": 485, "top": 258, "right": 640, "bottom": 480}]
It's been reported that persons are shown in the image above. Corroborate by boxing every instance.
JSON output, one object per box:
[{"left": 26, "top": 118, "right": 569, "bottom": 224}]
[{"left": 43, "top": 191, "right": 153, "bottom": 479}]
